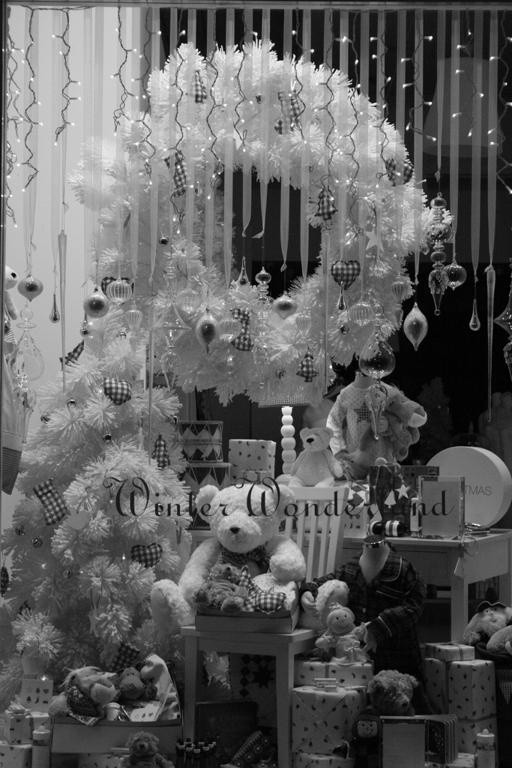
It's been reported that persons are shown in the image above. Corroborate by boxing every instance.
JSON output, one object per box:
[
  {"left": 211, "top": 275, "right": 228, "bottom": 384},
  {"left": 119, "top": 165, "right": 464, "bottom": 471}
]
[
  {"left": 326, "top": 375, "right": 427, "bottom": 483},
  {"left": 0, "top": 361, "right": 512, "bottom": 768},
  {"left": 303, "top": 533, "right": 429, "bottom": 702}
]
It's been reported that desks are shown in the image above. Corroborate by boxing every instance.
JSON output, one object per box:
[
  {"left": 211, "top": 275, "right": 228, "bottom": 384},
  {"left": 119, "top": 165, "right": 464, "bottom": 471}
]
[{"left": 183, "top": 527, "right": 511, "bottom": 649}]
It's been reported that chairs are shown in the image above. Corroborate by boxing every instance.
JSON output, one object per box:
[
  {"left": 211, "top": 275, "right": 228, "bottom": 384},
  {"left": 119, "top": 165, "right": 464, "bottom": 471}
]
[{"left": 176, "top": 483, "right": 349, "bottom": 768}]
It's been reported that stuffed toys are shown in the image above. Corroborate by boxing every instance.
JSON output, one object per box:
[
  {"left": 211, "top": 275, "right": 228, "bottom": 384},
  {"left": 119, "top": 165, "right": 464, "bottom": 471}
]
[
  {"left": 464, "top": 600, "right": 512, "bottom": 653},
  {"left": 117, "top": 731, "right": 176, "bottom": 768},
  {"left": 6, "top": 375, "right": 193, "bottom": 725},
  {"left": 311, "top": 578, "right": 419, "bottom": 717},
  {"left": 292, "top": 426, "right": 344, "bottom": 488},
  {"left": 177, "top": 483, "right": 306, "bottom": 617}
]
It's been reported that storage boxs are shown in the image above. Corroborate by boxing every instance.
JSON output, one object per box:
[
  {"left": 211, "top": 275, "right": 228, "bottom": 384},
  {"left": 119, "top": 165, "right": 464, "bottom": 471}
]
[{"left": 288, "top": 639, "right": 501, "bottom": 768}]
